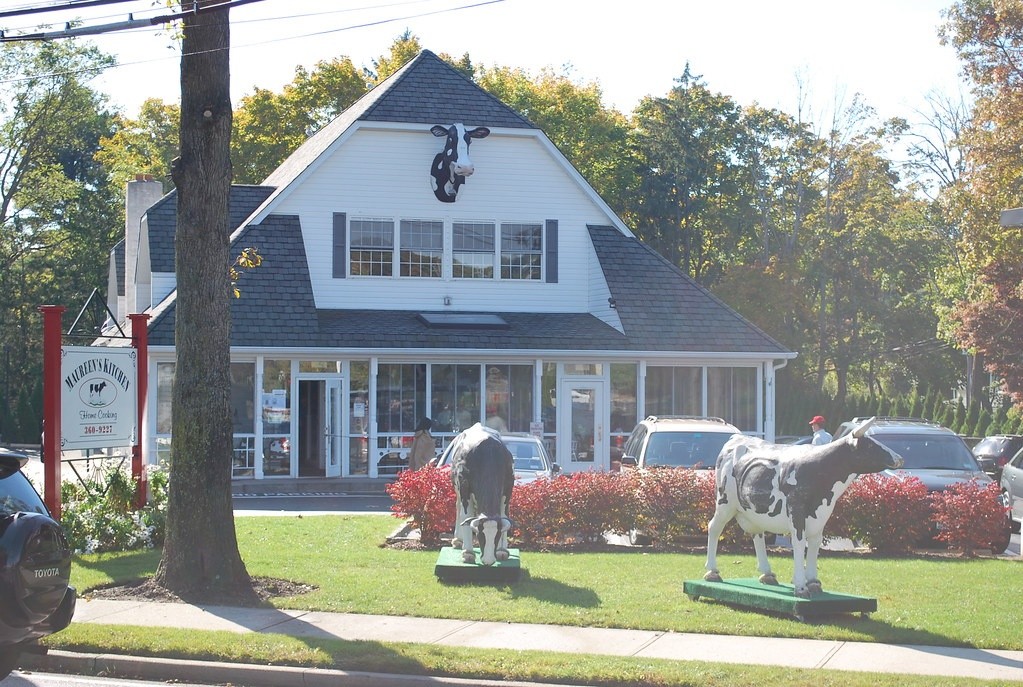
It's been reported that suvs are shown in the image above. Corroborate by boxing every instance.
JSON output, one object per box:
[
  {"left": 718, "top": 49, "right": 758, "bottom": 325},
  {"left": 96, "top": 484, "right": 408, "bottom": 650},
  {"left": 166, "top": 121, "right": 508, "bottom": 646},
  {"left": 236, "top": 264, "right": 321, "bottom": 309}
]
[
  {"left": 619, "top": 414, "right": 742, "bottom": 483},
  {"left": 588, "top": 421, "right": 636, "bottom": 461},
  {"left": 1, "top": 446, "right": 77, "bottom": 682},
  {"left": 827, "top": 417, "right": 1011, "bottom": 558}
]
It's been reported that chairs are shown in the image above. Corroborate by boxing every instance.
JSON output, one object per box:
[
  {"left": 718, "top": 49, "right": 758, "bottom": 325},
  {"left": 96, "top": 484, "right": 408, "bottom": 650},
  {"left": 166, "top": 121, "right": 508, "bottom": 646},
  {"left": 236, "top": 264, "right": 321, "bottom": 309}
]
[
  {"left": 516, "top": 444, "right": 533, "bottom": 462},
  {"left": 671, "top": 442, "right": 689, "bottom": 460}
]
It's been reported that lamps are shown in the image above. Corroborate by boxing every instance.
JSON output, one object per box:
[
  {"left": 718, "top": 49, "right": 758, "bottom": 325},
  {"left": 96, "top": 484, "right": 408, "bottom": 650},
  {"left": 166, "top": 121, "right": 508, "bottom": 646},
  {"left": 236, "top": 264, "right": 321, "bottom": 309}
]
[{"left": 608, "top": 298, "right": 617, "bottom": 309}]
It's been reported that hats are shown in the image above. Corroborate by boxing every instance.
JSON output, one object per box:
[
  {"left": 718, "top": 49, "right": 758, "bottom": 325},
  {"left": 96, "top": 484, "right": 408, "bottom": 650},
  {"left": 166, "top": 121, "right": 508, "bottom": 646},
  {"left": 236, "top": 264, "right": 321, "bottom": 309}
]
[{"left": 809, "top": 416, "right": 825, "bottom": 427}]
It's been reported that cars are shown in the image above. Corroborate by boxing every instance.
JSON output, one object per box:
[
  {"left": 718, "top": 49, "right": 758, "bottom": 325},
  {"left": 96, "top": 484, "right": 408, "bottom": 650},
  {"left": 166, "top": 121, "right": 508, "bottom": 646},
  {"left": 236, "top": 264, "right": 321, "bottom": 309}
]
[
  {"left": 431, "top": 430, "right": 565, "bottom": 488},
  {"left": 957, "top": 432, "right": 1023, "bottom": 534},
  {"left": 350, "top": 411, "right": 416, "bottom": 465},
  {"left": 236, "top": 418, "right": 289, "bottom": 473}
]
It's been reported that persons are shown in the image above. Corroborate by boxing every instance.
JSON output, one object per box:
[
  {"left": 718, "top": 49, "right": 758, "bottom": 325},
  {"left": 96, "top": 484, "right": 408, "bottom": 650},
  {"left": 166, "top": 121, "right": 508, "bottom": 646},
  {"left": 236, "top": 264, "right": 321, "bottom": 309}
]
[
  {"left": 458, "top": 401, "right": 471, "bottom": 431},
  {"left": 808, "top": 416, "right": 833, "bottom": 445},
  {"left": 409, "top": 417, "right": 434, "bottom": 470},
  {"left": 488, "top": 408, "right": 508, "bottom": 431},
  {"left": 437, "top": 408, "right": 452, "bottom": 432}
]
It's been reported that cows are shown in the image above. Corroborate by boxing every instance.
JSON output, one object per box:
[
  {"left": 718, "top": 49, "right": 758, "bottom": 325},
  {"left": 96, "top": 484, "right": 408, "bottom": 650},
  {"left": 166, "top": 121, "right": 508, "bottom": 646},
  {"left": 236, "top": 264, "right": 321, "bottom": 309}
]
[
  {"left": 449, "top": 421, "right": 516, "bottom": 566},
  {"left": 429, "top": 122, "right": 491, "bottom": 203},
  {"left": 703, "top": 416, "right": 905, "bottom": 598},
  {"left": 89, "top": 380, "right": 107, "bottom": 403}
]
